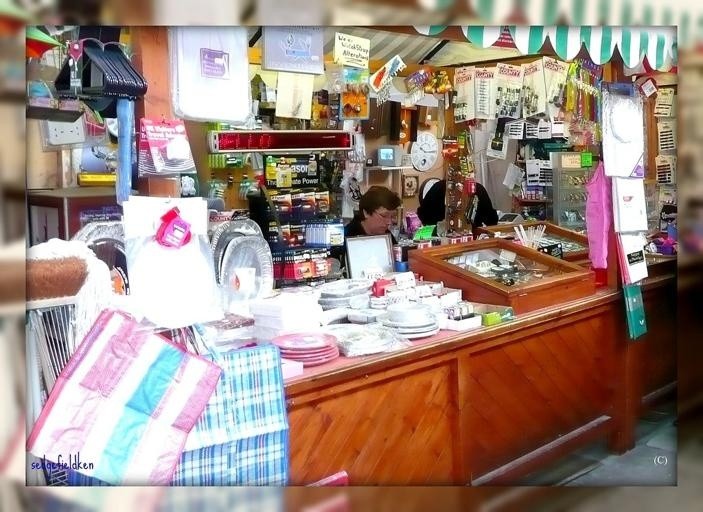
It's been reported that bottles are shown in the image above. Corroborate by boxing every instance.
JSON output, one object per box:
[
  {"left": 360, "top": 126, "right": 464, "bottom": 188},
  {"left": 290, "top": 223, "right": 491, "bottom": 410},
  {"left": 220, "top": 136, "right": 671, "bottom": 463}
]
[{"left": 254, "top": 115, "right": 263, "bottom": 131}]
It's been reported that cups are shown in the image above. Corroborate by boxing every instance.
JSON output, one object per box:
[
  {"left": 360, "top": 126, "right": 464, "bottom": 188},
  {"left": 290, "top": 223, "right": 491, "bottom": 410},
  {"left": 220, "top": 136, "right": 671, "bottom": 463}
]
[
  {"left": 370, "top": 279, "right": 391, "bottom": 297},
  {"left": 395, "top": 261, "right": 409, "bottom": 273}
]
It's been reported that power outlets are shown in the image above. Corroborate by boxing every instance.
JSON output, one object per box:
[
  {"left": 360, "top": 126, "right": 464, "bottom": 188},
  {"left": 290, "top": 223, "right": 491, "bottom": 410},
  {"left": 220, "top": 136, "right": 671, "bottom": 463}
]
[{"left": 45, "top": 117, "right": 86, "bottom": 146}]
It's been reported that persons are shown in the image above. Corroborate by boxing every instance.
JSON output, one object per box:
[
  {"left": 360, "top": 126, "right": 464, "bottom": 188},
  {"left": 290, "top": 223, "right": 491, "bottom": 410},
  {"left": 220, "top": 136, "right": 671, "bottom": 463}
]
[
  {"left": 345, "top": 186, "right": 402, "bottom": 244},
  {"left": 417, "top": 178, "right": 498, "bottom": 227}
]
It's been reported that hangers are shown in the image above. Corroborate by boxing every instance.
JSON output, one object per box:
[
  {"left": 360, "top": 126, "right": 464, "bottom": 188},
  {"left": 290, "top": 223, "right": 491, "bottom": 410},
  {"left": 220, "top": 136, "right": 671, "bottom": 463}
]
[{"left": 53, "top": 31, "right": 148, "bottom": 103}]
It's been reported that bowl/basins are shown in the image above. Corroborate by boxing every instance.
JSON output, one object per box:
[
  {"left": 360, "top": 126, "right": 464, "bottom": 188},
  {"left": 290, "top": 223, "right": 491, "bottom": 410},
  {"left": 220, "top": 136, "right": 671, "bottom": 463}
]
[{"left": 386, "top": 301, "right": 431, "bottom": 323}]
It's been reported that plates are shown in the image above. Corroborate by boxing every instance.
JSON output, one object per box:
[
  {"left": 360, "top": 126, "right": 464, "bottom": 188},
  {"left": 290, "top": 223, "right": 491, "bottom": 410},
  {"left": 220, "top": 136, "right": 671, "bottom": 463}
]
[
  {"left": 316, "top": 276, "right": 375, "bottom": 312},
  {"left": 209, "top": 215, "right": 276, "bottom": 300},
  {"left": 270, "top": 330, "right": 341, "bottom": 369},
  {"left": 376, "top": 313, "right": 442, "bottom": 341}
]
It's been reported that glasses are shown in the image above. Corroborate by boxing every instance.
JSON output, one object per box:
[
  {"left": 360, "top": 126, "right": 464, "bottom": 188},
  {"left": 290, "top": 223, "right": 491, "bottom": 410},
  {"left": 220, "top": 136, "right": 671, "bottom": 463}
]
[{"left": 374, "top": 211, "right": 397, "bottom": 220}]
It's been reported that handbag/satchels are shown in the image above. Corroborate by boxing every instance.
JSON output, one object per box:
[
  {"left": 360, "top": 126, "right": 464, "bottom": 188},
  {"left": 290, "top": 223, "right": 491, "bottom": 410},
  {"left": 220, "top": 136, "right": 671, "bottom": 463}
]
[{"left": 26, "top": 307, "right": 289, "bottom": 486}]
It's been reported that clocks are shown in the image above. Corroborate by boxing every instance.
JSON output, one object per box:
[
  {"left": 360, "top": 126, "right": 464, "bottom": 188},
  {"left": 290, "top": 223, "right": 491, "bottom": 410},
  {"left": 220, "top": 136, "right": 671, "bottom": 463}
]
[{"left": 411, "top": 132, "right": 440, "bottom": 172}]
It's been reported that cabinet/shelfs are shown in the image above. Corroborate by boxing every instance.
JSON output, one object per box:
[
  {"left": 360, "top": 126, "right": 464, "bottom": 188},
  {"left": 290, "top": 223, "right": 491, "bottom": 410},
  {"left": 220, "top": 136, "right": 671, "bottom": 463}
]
[{"left": 281, "top": 220, "right": 678, "bottom": 485}]
